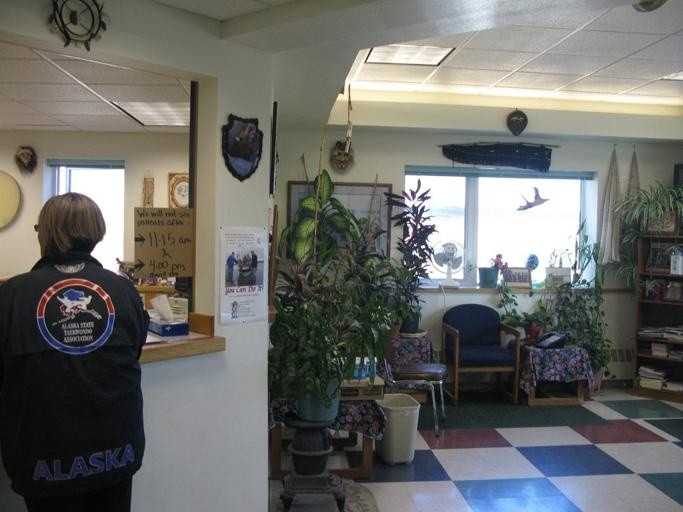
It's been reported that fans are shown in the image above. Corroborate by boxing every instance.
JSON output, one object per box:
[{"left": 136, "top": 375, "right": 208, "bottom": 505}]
[{"left": 430, "top": 239, "right": 467, "bottom": 290}]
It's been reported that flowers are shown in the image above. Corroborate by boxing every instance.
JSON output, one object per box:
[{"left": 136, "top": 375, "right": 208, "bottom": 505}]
[{"left": 491, "top": 254, "right": 510, "bottom": 276}]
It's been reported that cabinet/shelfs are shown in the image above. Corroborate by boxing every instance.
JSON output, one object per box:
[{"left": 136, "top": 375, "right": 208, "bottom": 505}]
[{"left": 633, "top": 236, "right": 682, "bottom": 401}]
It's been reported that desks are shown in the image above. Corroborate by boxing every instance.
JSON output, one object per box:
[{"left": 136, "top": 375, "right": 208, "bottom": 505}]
[
  {"left": 272, "top": 400, "right": 380, "bottom": 480},
  {"left": 510, "top": 341, "right": 588, "bottom": 407}
]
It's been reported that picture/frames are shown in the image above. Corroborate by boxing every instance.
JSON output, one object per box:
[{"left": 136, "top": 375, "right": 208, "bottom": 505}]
[
  {"left": 168, "top": 173, "right": 191, "bottom": 208},
  {"left": 288, "top": 180, "right": 392, "bottom": 262},
  {"left": 504, "top": 267, "right": 532, "bottom": 291},
  {"left": 644, "top": 206, "right": 679, "bottom": 235}
]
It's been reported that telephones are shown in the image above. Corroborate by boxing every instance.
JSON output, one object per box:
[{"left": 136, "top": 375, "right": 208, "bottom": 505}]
[{"left": 533, "top": 332, "right": 566, "bottom": 349}]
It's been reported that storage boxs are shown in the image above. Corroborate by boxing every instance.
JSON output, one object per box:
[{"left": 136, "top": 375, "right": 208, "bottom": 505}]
[{"left": 341, "top": 375, "right": 384, "bottom": 399}]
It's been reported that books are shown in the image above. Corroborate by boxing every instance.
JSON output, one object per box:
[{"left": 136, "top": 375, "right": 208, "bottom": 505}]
[{"left": 638, "top": 324, "right": 682, "bottom": 393}]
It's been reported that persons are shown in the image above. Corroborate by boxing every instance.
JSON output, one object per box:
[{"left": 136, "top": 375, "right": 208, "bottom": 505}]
[
  {"left": 227, "top": 250, "right": 257, "bottom": 282},
  {"left": 0, "top": 191, "right": 150, "bottom": 511}
]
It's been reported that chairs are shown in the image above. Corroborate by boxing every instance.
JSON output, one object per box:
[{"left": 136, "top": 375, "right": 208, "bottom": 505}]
[
  {"left": 387, "top": 359, "right": 449, "bottom": 436},
  {"left": 443, "top": 303, "right": 521, "bottom": 405}
]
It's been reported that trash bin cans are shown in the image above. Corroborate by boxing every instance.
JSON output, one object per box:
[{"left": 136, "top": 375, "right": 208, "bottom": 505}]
[{"left": 374, "top": 393, "right": 420, "bottom": 465}]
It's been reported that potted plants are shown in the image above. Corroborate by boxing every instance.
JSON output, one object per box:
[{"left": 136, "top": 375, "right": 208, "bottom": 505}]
[
  {"left": 548, "top": 218, "right": 610, "bottom": 395},
  {"left": 522, "top": 311, "right": 550, "bottom": 339},
  {"left": 275, "top": 180, "right": 433, "bottom": 422}
]
